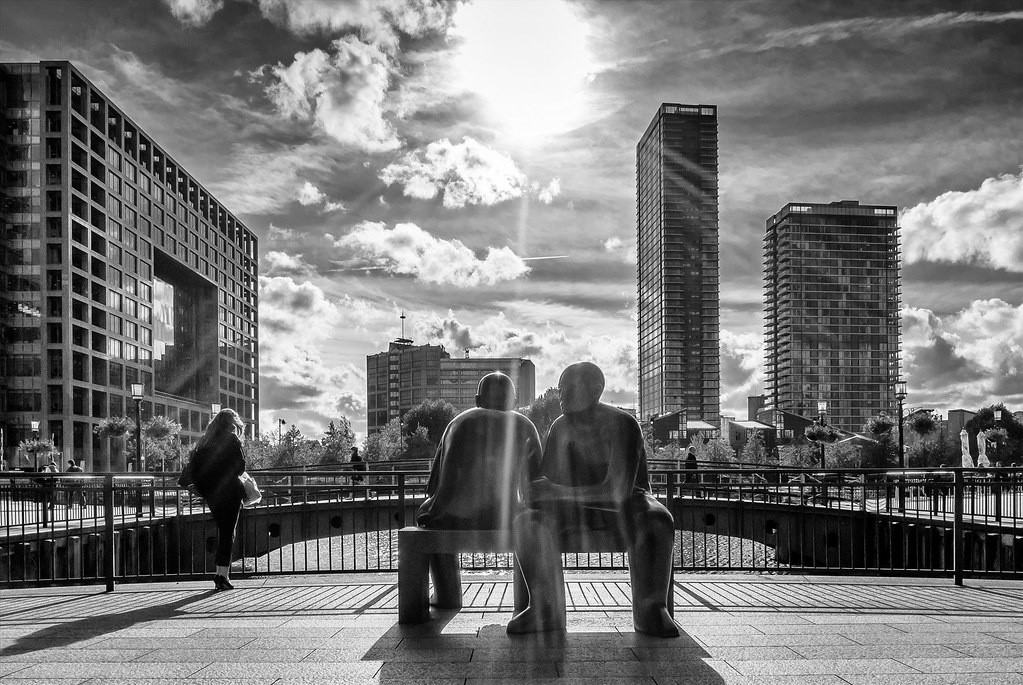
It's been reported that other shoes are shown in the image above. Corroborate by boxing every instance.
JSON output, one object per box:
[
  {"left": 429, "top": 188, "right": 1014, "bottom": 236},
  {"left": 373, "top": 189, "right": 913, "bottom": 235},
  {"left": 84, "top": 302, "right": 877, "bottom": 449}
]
[
  {"left": 346, "top": 497, "right": 352, "bottom": 500},
  {"left": 83, "top": 504, "right": 86, "bottom": 509},
  {"left": 66, "top": 505, "right": 72, "bottom": 509},
  {"left": 696, "top": 495, "right": 704, "bottom": 498},
  {"left": 48, "top": 506, "right": 55, "bottom": 510},
  {"left": 372, "top": 491, "right": 376, "bottom": 498},
  {"left": 680, "top": 494, "right": 684, "bottom": 497}
]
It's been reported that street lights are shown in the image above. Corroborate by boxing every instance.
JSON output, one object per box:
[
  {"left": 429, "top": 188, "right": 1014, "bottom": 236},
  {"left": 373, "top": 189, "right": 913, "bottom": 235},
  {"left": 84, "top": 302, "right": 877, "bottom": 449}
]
[
  {"left": 994, "top": 410, "right": 1002, "bottom": 466},
  {"left": 30, "top": 420, "right": 40, "bottom": 472},
  {"left": 399, "top": 419, "right": 404, "bottom": 452},
  {"left": 130, "top": 382, "right": 145, "bottom": 518},
  {"left": 816, "top": 400, "right": 828, "bottom": 506},
  {"left": 893, "top": 381, "right": 908, "bottom": 512}
]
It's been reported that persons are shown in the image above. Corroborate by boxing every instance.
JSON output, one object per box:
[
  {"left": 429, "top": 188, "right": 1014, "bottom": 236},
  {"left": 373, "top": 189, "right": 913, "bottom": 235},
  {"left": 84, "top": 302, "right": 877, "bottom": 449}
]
[
  {"left": 65, "top": 459, "right": 87, "bottom": 509},
  {"left": 996, "top": 462, "right": 1004, "bottom": 467},
  {"left": 346, "top": 447, "right": 376, "bottom": 500},
  {"left": 38, "top": 462, "right": 59, "bottom": 509},
  {"left": 975, "top": 463, "right": 987, "bottom": 476},
  {"left": 193, "top": 408, "right": 248, "bottom": 589},
  {"left": 506, "top": 362, "right": 680, "bottom": 638},
  {"left": 417, "top": 372, "right": 543, "bottom": 612},
  {"left": 677, "top": 446, "right": 705, "bottom": 498}
]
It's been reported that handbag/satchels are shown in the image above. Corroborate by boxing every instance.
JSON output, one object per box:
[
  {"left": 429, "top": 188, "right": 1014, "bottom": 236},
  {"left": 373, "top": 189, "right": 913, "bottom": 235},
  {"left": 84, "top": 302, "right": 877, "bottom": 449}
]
[
  {"left": 40, "top": 465, "right": 59, "bottom": 473},
  {"left": 238, "top": 471, "right": 262, "bottom": 507},
  {"left": 178, "top": 437, "right": 201, "bottom": 498}
]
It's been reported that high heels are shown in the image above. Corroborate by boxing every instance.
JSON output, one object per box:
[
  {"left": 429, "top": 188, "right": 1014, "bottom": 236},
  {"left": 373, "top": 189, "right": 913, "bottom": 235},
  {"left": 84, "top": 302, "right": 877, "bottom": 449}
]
[{"left": 214, "top": 575, "right": 234, "bottom": 591}]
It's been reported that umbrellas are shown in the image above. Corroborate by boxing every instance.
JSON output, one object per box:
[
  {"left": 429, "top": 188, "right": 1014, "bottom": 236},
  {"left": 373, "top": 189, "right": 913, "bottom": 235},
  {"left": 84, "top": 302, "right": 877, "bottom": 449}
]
[
  {"left": 959, "top": 429, "right": 974, "bottom": 468},
  {"left": 976, "top": 431, "right": 990, "bottom": 467}
]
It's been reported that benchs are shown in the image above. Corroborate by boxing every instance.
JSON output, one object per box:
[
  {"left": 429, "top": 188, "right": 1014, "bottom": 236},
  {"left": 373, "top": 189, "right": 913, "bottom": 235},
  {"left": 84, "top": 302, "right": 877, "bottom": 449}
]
[{"left": 397, "top": 525, "right": 676, "bottom": 627}]
